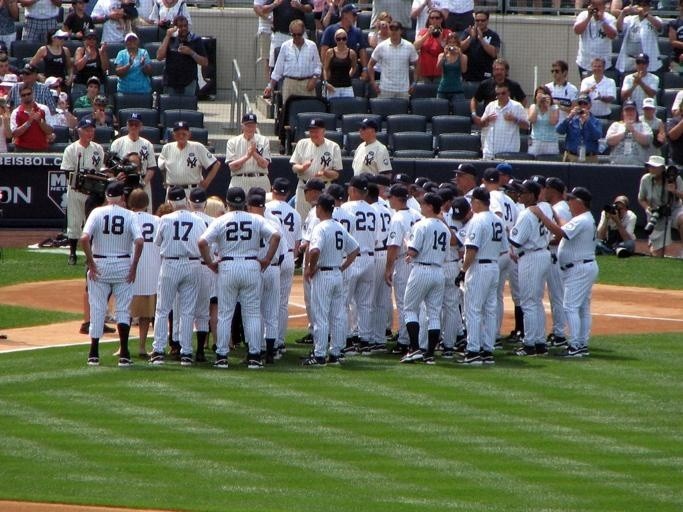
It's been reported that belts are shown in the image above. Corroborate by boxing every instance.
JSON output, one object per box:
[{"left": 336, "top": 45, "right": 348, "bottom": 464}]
[
  {"left": 93, "top": 252, "right": 132, "bottom": 259},
  {"left": 519, "top": 247, "right": 593, "bottom": 271},
  {"left": 165, "top": 248, "right": 295, "bottom": 266},
  {"left": 238, "top": 172, "right": 265, "bottom": 177},
  {"left": 320, "top": 247, "right": 387, "bottom": 271},
  {"left": 421, "top": 259, "right": 492, "bottom": 266},
  {"left": 285, "top": 75, "right": 313, "bottom": 80}
]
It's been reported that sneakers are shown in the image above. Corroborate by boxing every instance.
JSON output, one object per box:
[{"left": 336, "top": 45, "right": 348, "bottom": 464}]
[
  {"left": 507, "top": 331, "right": 592, "bottom": 358},
  {"left": 80, "top": 309, "right": 115, "bottom": 334},
  {"left": 294, "top": 333, "right": 503, "bottom": 367},
  {"left": 86, "top": 343, "right": 287, "bottom": 370},
  {"left": 68, "top": 253, "right": 78, "bottom": 266},
  {"left": 616, "top": 247, "right": 629, "bottom": 257}
]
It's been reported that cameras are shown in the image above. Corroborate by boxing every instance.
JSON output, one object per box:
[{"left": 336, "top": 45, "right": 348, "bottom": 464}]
[
  {"left": 576, "top": 109, "right": 584, "bottom": 114},
  {"left": 448, "top": 44, "right": 455, "bottom": 51},
  {"left": 603, "top": 204, "right": 621, "bottom": 215},
  {"left": 644, "top": 207, "right": 671, "bottom": 234},
  {"left": 541, "top": 94, "right": 547, "bottom": 102},
  {"left": 431, "top": 25, "right": 442, "bottom": 38},
  {"left": 637, "top": 8, "right": 644, "bottom": 15},
  {"left": 592, "top": 8, "right": 598, "bottom": 14}
]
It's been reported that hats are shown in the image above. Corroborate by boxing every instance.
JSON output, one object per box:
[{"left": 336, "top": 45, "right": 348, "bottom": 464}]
[
  {"left": 95, "top": 94, "right": 107, "bottom": 103},
  {"left": 358, "top": 117, "right": 377, "bottom": 128},
  {"left": 300, "top": 163, "right": 594, "bottom": 220},
  {"left": 242, "top": 114, "right": 257, "bottom": 124},
  {"left": 106, "top": 183, "right": 123, "bottom": 198},
  {"left": 2, "top": 30, "right": 139, "bottom": 87},
  {"left": 623, "top": 53, "right": 656, "bottom": 110},
  {"left": 78, "top": 119, "right": 96, "bottom": 130},
  {"left": 615, "top": 194, "right": 629, "bottom": 207},
  {"left": 644, "top": 156, "right": 665, "bottom": 169},
  {"left": 389, "top": 21, "right": 402, "bottom": 28},
  {"left": 308, "top": 118, "right": 325, "bottom": 130},
  {"left": 341, "top": 4, "right": 361, "bottom": 14},
  {"left": 173, "top": 122, "right": 190, "bottom": 132},
  {"left": 168, "top": 178, "right": 292, "bottom": 207},
  {"left": 577, "top": 93, "right": 591, "bottom": 105},
  {"left": 127, "top": 112, "right": 142, "bottom": 122}
]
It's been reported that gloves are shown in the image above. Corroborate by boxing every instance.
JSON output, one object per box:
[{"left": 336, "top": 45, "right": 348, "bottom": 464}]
[{"left": 454, "top": 270, "right": 466, "bottom": 286}]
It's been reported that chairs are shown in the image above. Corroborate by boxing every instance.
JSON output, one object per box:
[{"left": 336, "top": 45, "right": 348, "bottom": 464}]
[{"left": 0, "top": 0, "right": 683, "bottom": 166}]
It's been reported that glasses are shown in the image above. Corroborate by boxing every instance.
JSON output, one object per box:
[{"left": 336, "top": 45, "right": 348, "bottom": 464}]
[
  {"left": 475, "top": 19, "right": 485, "bottom": 22},
  {"left": 337, "top": 37, "right": 348, "bottom": 41},
  {"left": 430, "top": 16, "right": 441, "bottom": 20},
  {"left": 21, "top": 92, "right": 31, "bottom": 96},
  {"left": 291, "top": 32, "right": 302, "bottom": 37},
  {"left": 551, "top": 69, "right": 559, "bottom": 73},
  {"left": 495, "top": 92, "right": 506, "bottom": 96}
]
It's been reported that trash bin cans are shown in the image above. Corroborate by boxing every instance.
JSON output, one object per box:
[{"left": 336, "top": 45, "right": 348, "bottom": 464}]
[{"left": 196, "top": 35, "right": 217, "bottom": 102}]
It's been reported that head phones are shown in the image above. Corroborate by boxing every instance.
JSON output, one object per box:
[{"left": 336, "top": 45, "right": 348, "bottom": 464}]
[{"left": 122, "top": 152, "right": 138, "bottom": 165}]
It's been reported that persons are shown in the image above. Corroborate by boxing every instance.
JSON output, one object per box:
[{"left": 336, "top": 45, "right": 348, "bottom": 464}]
[
  {"left": 0, "top": 0, "right": 210, "bottom": 154},
  {"left": 60, "top": 112, "right": 219, "bottom": 265},
  {"left": 78, "top": 178, "right": 302, "bottom": 370},
  {"left": 289, "top": 118, "right": 392, "bottom": 270},
  {"left": 469, "top": 58, "right": 683, "bottom": 165},
  {"left": 297, "top": 162, "right": 599, "bottom": 366},
  {"left": 251, "top": 0, "right": 499, "bottom": 102},
  {"left": 226, "top": 113, "right": 271, "bottom": 196},
  {"left": 593, "top": 154, "right": 683, "bottom": 258},
  {"left": 574, "top": 2, "right": 683, "bottom": 92}
]
